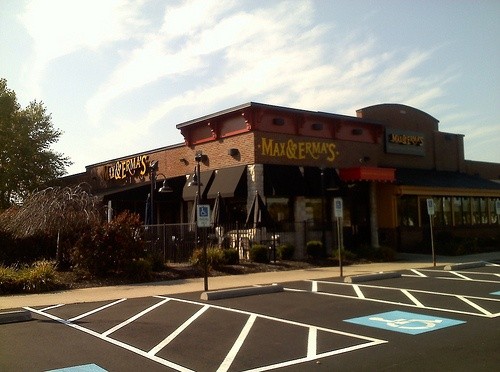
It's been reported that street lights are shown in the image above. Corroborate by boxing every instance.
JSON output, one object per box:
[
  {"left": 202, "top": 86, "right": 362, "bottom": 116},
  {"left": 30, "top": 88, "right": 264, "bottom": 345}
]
[
  {"left": 147, "top": 163, "right": 174, "bottom": 274},
  {"left": 320, "top": 164, "right": 342, "bottom": 264},
  {"left": 186, "top": 156, "right": 212, "bottom": 292}
]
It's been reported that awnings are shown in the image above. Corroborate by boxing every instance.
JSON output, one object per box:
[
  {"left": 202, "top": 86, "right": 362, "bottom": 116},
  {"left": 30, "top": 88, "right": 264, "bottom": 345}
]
[
  {"left": 206, "top": 165, "right": 247, "bottom": 199},
  {"left": 302, "top": 166, "right": 341, "bottom": 193},
  {"left": 340, "top": 167, "right": 398, "bottom": 183},
  {"left": 178, "top": 169, "right": 213, "bottom": 203},
  {"left": 264, "top": 164, "right": 307, "bottom": 197}
]
